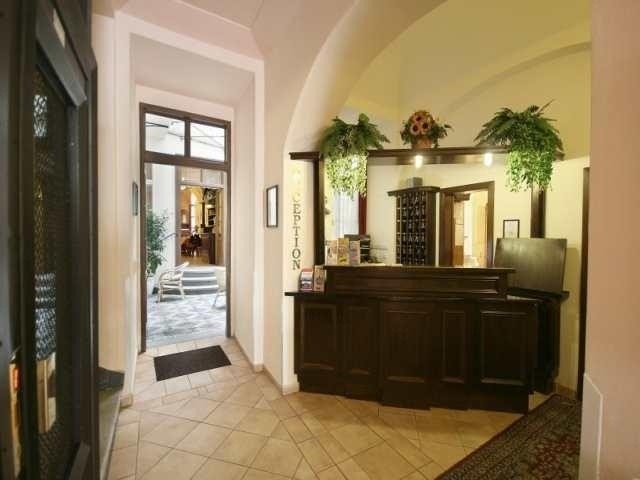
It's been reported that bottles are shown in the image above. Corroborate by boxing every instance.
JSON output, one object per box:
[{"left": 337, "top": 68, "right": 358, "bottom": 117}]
[{"left": 396, "top": 192, "right": 429, "bottom": 266}]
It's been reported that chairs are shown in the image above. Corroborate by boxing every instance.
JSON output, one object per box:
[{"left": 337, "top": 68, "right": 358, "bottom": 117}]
[
  {"left": 211, "top": 267, "right": 227, "bottom": 308},
  {"left": 156, "top": 262, "right": 190, "bottom": 302}
]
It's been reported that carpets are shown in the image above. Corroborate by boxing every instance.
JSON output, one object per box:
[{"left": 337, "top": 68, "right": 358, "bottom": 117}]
[
  {"left": 152, "top": 344, "right": 233, "bottom": 382},
  {"left": 431, "top": 392, "right": 581, "bottom": 480}
]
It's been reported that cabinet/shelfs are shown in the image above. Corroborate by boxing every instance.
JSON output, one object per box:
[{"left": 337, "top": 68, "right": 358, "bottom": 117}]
[{"left": 387, "top": 186, "right": 440, "bottom": 266}]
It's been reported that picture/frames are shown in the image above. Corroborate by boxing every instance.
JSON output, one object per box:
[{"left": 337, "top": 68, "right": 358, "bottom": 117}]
[
  {"left": 266, "top": 184, "right": 278, "bottom": 228},
  {"left": 131, "top": 182, "right": 139, "bottom": 217},
  {"left": 502, "top": 219, "right": 520, "bottom": 239}
]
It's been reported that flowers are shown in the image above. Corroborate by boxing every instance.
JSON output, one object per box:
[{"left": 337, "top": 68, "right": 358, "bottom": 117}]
[{"left": 399, "top": 110, "right": 453, "bottom": 149}]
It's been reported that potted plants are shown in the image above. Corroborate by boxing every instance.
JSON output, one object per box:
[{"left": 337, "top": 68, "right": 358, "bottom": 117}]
[{"left": 145, "top": 207, "right": 169, "bottom": 298}]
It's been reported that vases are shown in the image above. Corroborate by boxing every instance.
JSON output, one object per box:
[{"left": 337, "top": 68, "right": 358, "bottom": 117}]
[{"left": 411, "top": 134, "right": 432, "bottom": 150}]
